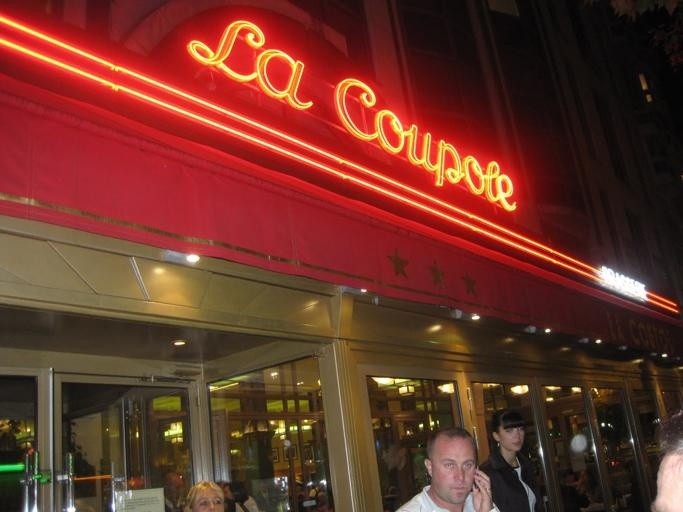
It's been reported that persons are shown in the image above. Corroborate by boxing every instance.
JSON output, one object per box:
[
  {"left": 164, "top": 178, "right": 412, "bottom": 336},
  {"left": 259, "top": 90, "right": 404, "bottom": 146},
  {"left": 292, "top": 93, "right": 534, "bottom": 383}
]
[
  {"left": 157, "top": 469, "right": 259, "bottom": 512},
  {"left": 391, "top": 423, "right": 501, "bottom": 512},
  {"left": 379, "top": 432, "right": 425, "bottom": 512},
  {"left": 474, "top": 407, "right": 541, "bottom": 511},
  {"left": 648, "top": 411, "right": 682, "bottom": 512},
  {"left": 529, "top": 455, "right": 619, "bottom": 511}
]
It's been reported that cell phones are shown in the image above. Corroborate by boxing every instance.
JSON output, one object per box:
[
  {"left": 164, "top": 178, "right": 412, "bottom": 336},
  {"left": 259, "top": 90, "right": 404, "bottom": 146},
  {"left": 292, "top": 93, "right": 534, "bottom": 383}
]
[{"left": 473, "top": 464, "right": 481, "bottom": 489}]
